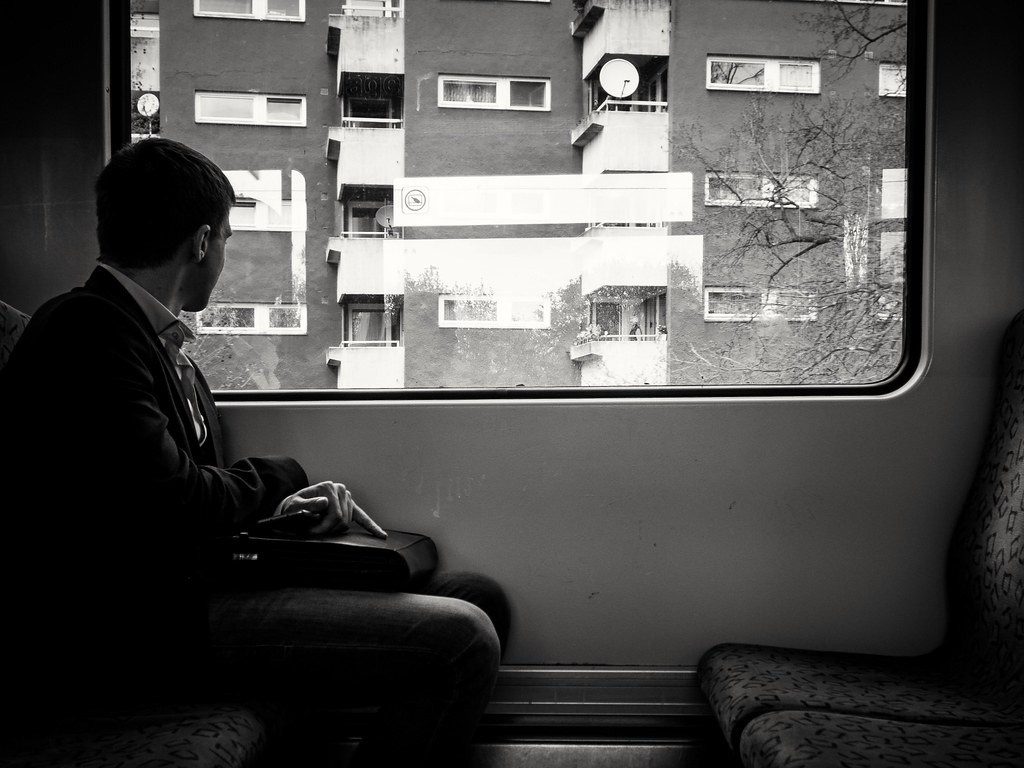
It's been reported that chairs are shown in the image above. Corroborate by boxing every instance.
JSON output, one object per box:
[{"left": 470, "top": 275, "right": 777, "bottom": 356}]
[
  {"left": 0, "top": 288, "right": 280, "bottom": 768},
  {"left": 698, "top": 308, "right": 1024, "bottom": 768}
]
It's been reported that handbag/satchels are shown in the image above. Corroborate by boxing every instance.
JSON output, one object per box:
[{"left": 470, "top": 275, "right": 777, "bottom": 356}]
[{"left": 233, "top": 510, "right": 438, "bottom": 587}]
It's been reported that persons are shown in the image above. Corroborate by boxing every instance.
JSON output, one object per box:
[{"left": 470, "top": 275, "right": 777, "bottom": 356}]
[{"left": 0, "top": 140, "right": 510, "bottom": 767}]
